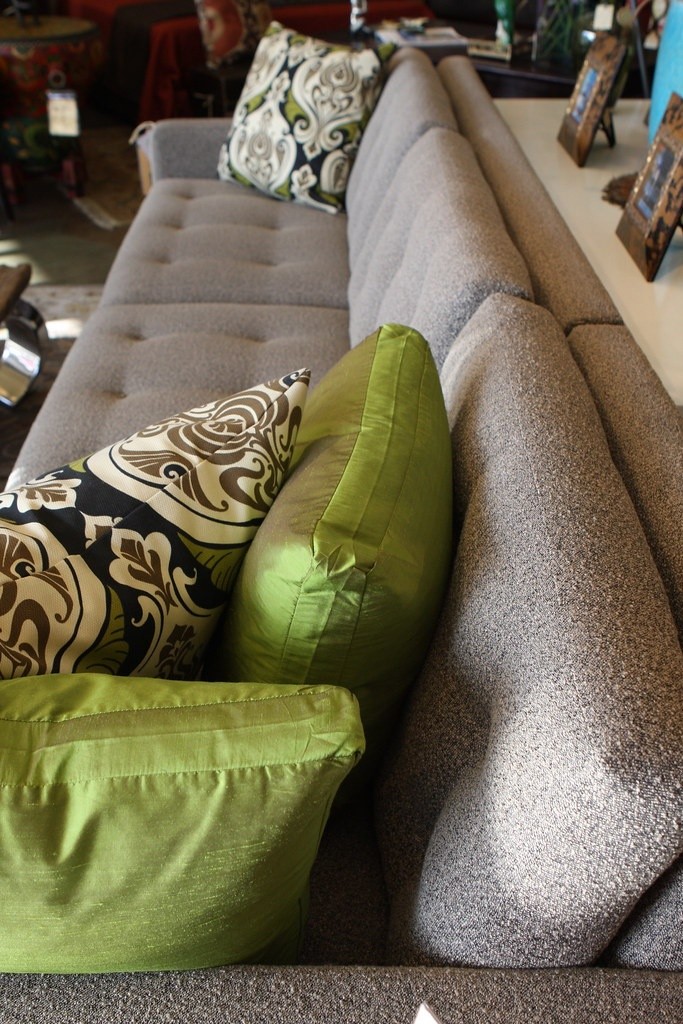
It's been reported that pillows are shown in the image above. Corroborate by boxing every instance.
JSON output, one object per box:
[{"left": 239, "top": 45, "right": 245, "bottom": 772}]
[
  {"left": 234, "top": 323, "right": 452, "bottom": 699},
  {"left": 1, "top": 672, "right": 368, "bottom": 972},
  {"left": 216, "top": 19, "right": 380, "bottom": 217},
  {"left": 0, "top": 368, "right": 315, "bottom": 683}
]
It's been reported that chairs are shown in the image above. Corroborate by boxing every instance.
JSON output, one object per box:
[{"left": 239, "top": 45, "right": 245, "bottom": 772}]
[
  {"left": 1, "top": 71, "right": 85, "bottom": 210},
  {"left": 142, "top": 17, "right": 209, "bottom": 120}
]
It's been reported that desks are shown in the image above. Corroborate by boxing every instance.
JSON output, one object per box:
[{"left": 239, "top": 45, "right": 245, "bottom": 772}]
[
  {"left": 466, "top": 55, "right": 577, "bottom": 86},
  {"left": 495, "top": 98, "right": 683, "bottom": 410},
  {"left": 1, "top": 15, "right": 104, "bottom": 197}
]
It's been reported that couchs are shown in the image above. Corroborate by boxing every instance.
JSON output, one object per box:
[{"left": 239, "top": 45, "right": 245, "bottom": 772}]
[{"left": 0, "top": 55, "right": 683, "bottom": 1024}]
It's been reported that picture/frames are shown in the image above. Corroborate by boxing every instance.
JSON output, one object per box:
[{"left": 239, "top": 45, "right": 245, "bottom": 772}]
[
  {"left": 558, "top": 31, "right": 636, "bottom": 167},
  {"left": 615, "top": 90, "right": 683, "bottom": 283}
]
